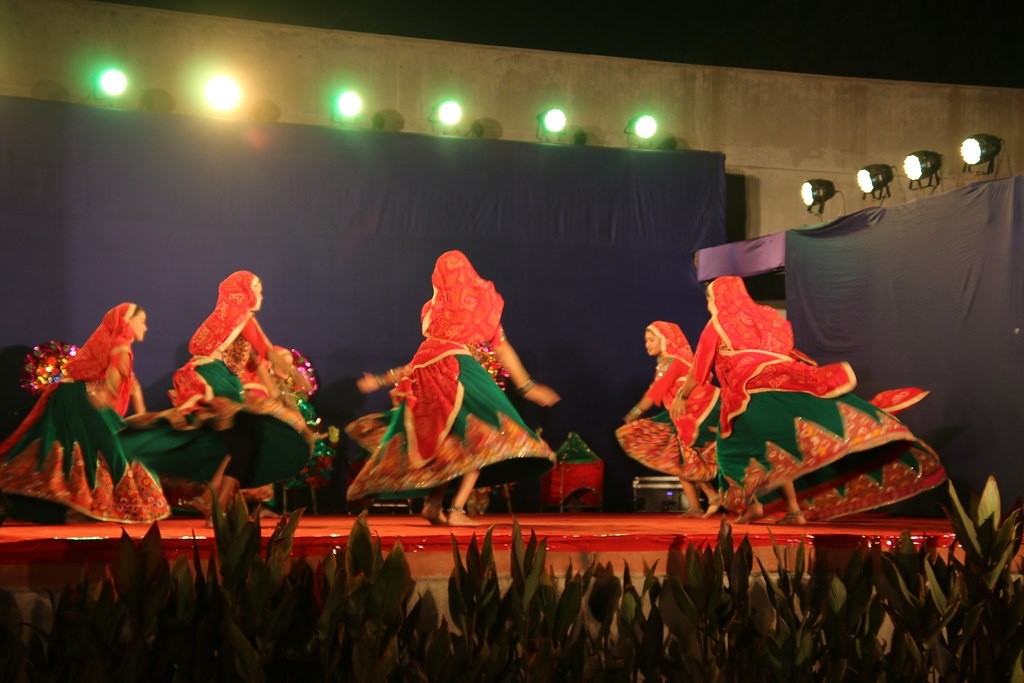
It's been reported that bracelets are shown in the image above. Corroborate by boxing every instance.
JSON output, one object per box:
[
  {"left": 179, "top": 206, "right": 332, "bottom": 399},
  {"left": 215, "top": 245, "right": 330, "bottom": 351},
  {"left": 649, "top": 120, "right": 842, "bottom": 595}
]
[
  {"left": 677, "top": 389, "right": 688, "bottom": 400},
  {"left": 630, "top": 407, "right": 642, "bottom": 417},
  {"left": 520, "top": 378, "right": 537, "bottom": 396}
]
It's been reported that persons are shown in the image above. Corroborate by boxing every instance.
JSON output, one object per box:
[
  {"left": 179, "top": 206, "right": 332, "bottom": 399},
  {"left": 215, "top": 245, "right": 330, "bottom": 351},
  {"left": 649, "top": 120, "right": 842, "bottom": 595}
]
[
  {"left": 0, "top": 252, "right": 560, "bottom": 527},
  {"left": 615, "top": 321, "right": 724, "bottom": 518},
  {"left": 669, "top": 276, "right": 947, "bottom": 526}
]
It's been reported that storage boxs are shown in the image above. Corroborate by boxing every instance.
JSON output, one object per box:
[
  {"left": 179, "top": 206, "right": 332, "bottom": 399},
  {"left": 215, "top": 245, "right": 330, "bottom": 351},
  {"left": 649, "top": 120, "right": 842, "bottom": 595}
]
[{"left": 634, "top": 469, "right": 702, "bottom": 513}]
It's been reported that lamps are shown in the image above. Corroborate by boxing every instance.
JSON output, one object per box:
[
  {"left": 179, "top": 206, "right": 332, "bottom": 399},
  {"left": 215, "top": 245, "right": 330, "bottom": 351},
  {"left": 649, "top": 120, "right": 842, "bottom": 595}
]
[
  {"left": 801, "top": 179, "right": 845, "bottom": 222},
  {"left": 960, "top": 133, "right": 1002, "bottom": 174},
  {"left": 857, "top": 164, "right": 894, "bottom": 201},
  {"left": 902, "top": 150, "right": 944, "bottom": 189}
]
